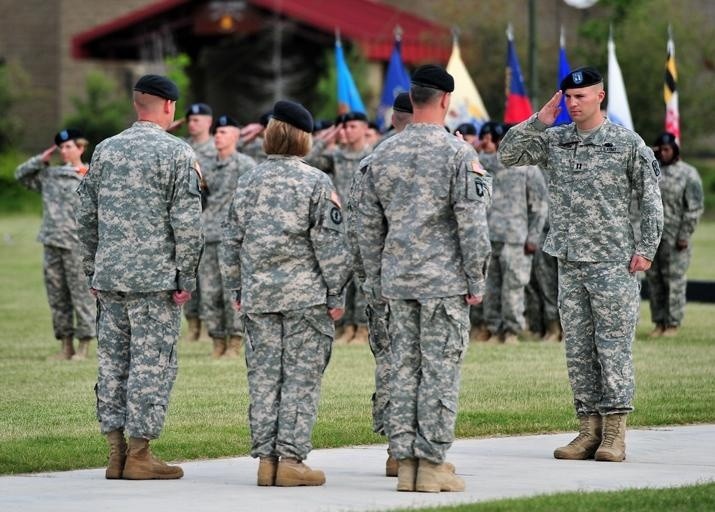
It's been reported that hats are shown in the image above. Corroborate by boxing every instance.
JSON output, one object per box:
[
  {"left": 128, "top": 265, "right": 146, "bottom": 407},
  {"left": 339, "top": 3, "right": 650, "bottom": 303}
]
[
  {"left": 559, "top": 66, "right": 602, "bottom": 92},
  {"left": 314, "top": 113, "right": 372, "bottom": 133},
  {"left": 454, "top": 122, "right": 515, "bottom": 142},
  {"left": 393, "top": 65, "right": 454, "bottom": 113},
  {"left": 655, "top": 132, "right": 675, "bottom": 146},
  {"left": 134, "top": 75, "right": 179, "bottom": 101},
  {"left": 213, "top": 115, "right": 241, "bottom": 128},
  {"left": 54, "top": 128, "right": 85, "bottom": 146},
  {"left": 273, "top": 101, "right": 314, "bottom": 133},
  {"left": 187, "top": 102, "right": 213, "bottom": 116}
]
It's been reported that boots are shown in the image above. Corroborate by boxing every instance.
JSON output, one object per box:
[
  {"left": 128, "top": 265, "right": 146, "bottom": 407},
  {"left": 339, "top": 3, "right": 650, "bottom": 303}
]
[
  {"left": 553, "top": 410, "right": 602, "bottom": 460},
  {"left": 258, "top": 456, "right": 325, "bottom": 487},
  {"left": 331, "top": 320, "right": 370, "bottom": 346},
  {"left": 177, "top": 315, "right": 243, "bottom": 360},
  {"left": 477, "top": 319, "right": 562, "bottom": 344},
  {"left": 594, "top": 409, "right": 628, "bottom": 459},
  {"left": 69, "top": 338, "right": 90, "bottom": 360},
  {"left": 386, "top": 453, "right": 467, "bottom": 491},
  {"left": 45, "top": 335, "right": 75, "bottom": 361},
  {"left": 105, "top": 426, "right": 183, "bottom": 479},
  {"left": 651, "top": 325, "right": 677, "bottom": 337}
]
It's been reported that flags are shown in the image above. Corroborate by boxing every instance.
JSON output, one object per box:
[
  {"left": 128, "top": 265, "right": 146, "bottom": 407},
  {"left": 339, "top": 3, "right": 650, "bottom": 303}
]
[{"left": 328, "top": 28, "right": 682, "bottom": 153}]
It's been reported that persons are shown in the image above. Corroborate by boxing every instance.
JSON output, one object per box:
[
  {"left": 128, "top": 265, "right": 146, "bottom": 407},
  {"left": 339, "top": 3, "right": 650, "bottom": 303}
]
[
  {"left": 347, "top": 65, "right": 491, "bottom": 494},
  {"left": 163, "top": 103, "right": 705, "bottom": 356},
  {"left": 76, "top": 72, "right": 206, "bottom": 478},
  {"left": 14, "top": 129, "right": 95, "bottom": 362},
  {"left": 497, "top": 68, "right": 665, "bottom": 463},
  {"left": 217, "top": 100, "right": 347, "bottom": 486},
  {"left": 349, "top": 92, "right": 456, "bottom": 478}
]
